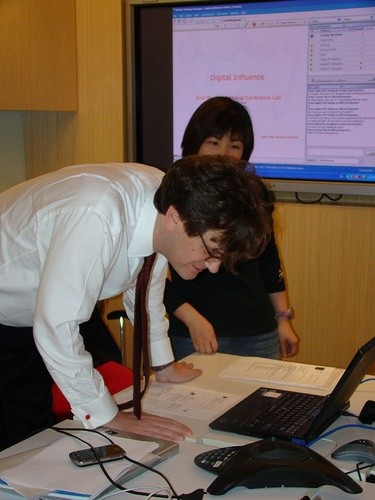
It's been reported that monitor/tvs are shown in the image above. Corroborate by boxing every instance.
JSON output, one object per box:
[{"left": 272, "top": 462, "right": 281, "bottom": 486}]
[{"left": 131, "top": 0, "right": 375, "bottom": 194}]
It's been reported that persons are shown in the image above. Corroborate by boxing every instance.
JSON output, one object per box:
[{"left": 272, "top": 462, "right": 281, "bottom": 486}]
[
  {"left": 0, "top": 156, "right": 278, "bottom": 452},
  {"left": 162, "top": 96, "right": 299, "bottom": 361}
]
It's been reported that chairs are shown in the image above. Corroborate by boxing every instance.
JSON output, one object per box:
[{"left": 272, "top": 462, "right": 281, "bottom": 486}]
[{"left": 52, "top": 309, "right": 134, "bottom": 417}]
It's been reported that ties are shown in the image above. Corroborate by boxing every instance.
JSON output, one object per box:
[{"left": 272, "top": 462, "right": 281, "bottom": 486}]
[{"left": 117, "top": 253, "right": 158, "bottom": 420}]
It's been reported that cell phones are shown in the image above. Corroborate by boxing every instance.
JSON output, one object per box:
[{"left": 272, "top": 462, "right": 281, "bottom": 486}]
[{"left": 69, "top": 444, "right": 126, "bottom": 468}]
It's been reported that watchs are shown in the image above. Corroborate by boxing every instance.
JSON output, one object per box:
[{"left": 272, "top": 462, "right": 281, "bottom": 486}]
[{"left": 274, "top": 305, "right": 295, "bottom": 321}]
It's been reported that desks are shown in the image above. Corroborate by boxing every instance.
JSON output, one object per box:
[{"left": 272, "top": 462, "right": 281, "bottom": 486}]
[{"left": 0, "top": 350, "right": 375, "bottom": 500}]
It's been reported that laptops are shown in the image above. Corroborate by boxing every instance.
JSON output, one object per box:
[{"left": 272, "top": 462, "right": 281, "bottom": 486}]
[
  {"left": 209, "top": 339, "right": 375, "bottom": 444},
  {"left": 0, "top": 425, "right": 180, "bottom": 499}
]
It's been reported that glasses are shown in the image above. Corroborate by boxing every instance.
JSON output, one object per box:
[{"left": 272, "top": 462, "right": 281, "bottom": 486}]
[{"left": 199, "top": 233, "right": 224, "bottom": 262}]
